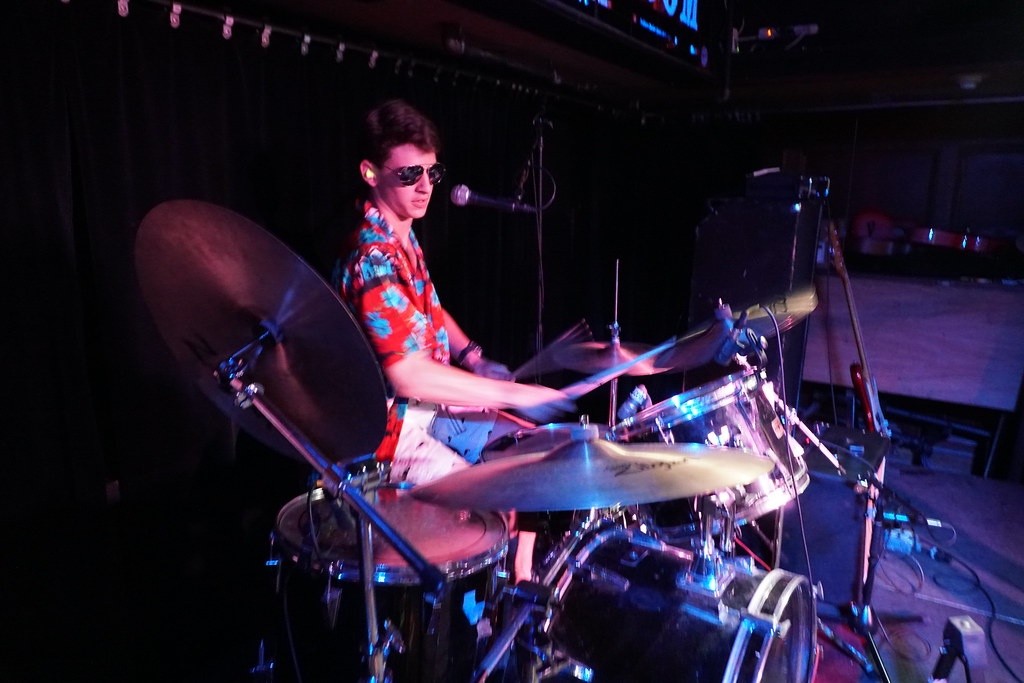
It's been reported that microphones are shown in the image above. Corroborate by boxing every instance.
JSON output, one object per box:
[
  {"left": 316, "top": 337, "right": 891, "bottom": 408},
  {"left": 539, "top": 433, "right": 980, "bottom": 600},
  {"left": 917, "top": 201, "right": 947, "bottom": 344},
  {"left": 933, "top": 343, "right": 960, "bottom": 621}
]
[
  {"left": 618, "top": 393, "right": 645, "bottom": 419},
  {"left": 451, "top": 185, "right": 538, "bottom": 214},
  {"left": 714, "top": 311, "right": 749, "bottom": 365}
]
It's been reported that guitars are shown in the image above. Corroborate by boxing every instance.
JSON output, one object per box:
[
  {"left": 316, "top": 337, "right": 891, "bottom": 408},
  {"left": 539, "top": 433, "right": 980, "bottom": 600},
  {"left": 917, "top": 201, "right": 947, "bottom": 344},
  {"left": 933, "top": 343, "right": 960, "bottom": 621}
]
[{"left": 825, "top": 224, "right": 893, "bottom": 440}]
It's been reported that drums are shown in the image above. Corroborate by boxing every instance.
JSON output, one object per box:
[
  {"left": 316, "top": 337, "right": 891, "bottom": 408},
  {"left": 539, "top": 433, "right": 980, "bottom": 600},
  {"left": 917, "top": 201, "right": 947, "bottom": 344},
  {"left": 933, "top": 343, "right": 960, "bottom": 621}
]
[
  {"left": 603, "top": 370, "right": 813, "bottom": 537},
  {"left": 524, "top": 514, "right": 819, "bottom": 683},
  {"left": 260, "top": 481, "right": 513, "bottom": 683},
  {"left": 480, "top": 420, "right": 609, "bottom": 464}
]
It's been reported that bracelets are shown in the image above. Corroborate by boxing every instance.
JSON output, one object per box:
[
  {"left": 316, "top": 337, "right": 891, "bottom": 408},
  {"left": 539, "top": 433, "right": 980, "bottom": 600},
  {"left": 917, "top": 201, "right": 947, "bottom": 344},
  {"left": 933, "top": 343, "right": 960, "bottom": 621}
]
[{"left": 456, "top": 340, "right": 482, "bottom": 367}]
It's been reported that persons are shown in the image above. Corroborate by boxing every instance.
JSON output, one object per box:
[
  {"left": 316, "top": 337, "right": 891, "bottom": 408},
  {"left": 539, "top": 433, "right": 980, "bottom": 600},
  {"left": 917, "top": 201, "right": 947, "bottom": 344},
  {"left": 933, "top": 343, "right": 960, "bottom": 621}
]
[{"left": 325, "top": 100, "right": 576, "bottom": 584}]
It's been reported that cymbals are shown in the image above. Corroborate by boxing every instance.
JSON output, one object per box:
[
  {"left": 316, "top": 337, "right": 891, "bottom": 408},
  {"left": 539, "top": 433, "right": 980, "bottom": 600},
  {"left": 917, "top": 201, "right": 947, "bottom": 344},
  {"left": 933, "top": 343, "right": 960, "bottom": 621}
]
[
  {"left": 408, "top": 440, "right": 778, "bottom": 512},
  {"left": 654, "top": 283, "right": 819, "bottom": 370},
  {"left": 136, "top": 198, "right": 391, "bottom": 459},
  {"left": 553, "top": 343, "right": 677, "bottom": 376}
]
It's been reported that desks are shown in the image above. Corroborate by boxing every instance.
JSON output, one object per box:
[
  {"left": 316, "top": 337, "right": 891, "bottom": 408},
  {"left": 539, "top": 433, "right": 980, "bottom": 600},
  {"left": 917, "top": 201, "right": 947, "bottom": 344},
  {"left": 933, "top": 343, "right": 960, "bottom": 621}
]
[{"left": 806, "top": 278, "right": 1024, "bottom": 480}]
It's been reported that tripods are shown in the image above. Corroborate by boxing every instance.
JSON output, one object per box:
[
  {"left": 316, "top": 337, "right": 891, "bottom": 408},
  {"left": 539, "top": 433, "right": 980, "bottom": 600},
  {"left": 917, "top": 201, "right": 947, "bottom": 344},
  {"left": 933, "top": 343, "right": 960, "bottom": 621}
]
[{"left": 815, "top": 469, "right": 931, "bottom": 683}]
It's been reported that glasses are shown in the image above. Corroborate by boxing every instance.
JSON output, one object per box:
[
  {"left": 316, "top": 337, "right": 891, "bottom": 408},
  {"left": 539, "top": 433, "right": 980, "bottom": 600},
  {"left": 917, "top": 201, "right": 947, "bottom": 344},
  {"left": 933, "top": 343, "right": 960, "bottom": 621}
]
[{"left": 375, "top": 162, "right": 447, "bottom": 187}]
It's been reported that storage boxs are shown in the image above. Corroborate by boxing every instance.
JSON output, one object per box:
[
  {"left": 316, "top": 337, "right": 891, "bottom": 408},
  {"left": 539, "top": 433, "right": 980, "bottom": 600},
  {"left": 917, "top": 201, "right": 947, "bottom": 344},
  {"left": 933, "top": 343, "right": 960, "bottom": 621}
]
[{"left": 893, "top": 424, "right": 976, "bottom": 476}]
